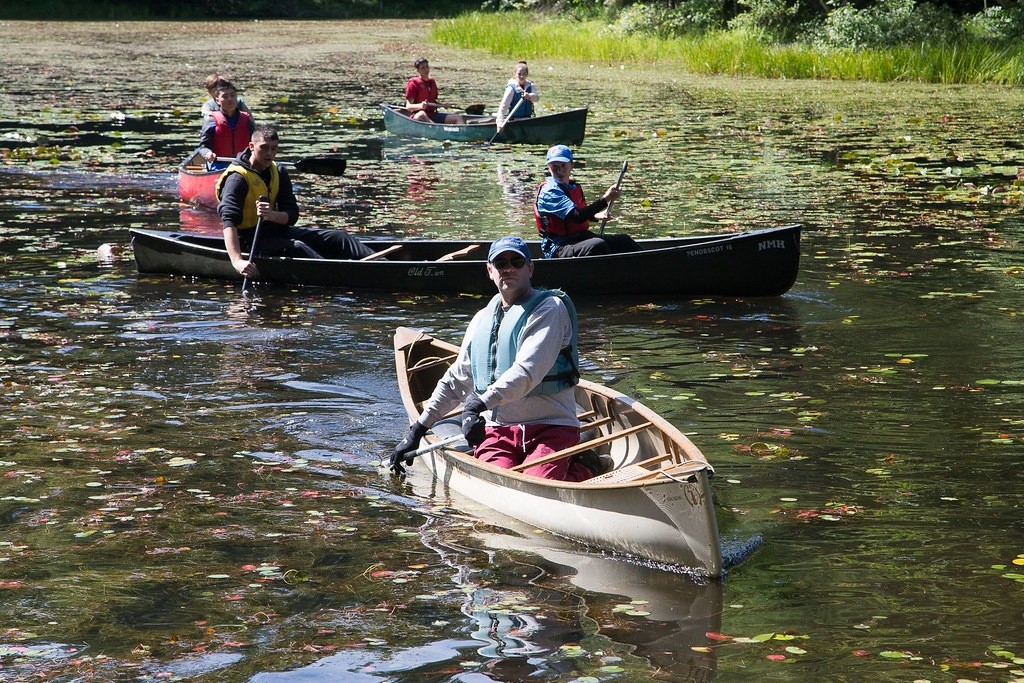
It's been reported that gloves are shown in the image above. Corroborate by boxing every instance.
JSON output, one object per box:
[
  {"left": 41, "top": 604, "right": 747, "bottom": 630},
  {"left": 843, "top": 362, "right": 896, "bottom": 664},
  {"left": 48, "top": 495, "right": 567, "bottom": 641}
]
[
  {"left": 460, "top": 397, "right": 485, "bottom": 449},
  {"left": 391, "top": 422, "right": 428, "bottom": 473}
]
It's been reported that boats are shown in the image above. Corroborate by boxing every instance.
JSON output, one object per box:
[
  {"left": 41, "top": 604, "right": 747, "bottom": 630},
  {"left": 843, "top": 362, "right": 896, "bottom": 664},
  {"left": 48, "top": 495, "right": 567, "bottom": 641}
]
[
  {"left": 129, "top": 222, "right": 805, "bottom": 298},
  {"left": 175, "top": 146, "right": 228, "bottom": 212},
  {"left": 391, "top": 323, "right": 724, "bottom": 580},
  {"left": 376, "top": 101, "right": 588, "bottom": 144}
]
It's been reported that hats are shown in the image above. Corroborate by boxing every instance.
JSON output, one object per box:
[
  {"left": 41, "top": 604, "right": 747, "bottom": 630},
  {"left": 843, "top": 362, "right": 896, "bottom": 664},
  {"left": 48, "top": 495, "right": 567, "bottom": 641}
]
[
  {"left": 488, "top": 235, "right": 531, "bottom": 264},
  {"left": 546, "top": 145, "right": 573, "bottom": 164}
]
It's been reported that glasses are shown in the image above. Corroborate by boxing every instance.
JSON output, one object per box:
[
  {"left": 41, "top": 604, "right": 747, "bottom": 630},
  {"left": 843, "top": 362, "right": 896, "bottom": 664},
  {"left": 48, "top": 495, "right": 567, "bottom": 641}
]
[
  {"left": 548, "top": 162, "right": 567, "bottom": 170},
  {"left": 492, "top": 255, "right": 526, "bottom": 270}
]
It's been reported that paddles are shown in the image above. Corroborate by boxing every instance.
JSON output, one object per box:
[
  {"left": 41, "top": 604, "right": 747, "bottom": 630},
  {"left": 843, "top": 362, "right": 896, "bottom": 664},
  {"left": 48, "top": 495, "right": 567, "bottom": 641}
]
[
  {"left": 382, "top": 434, "right": 464, "bottom": 466},
  {"left": 214, "top": 157, "right": 347, "bottom": 176},
  {"left": 437, "top": 104, "right": 487, "bottom": 115},
  {"left": 241, "top": 195, "right": 271, "bottom": 289},
  {"left": 488, "top": 97, "right": 525, "bottom": 144},
  {"left": 599, "top": 158, "right": 629, "bottom": 234}
]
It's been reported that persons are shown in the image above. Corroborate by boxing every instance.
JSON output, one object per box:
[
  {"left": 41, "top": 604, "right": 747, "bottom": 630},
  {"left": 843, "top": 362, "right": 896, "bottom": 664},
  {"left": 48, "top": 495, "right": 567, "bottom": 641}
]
[
  {"left": 391, "top": 235, "right": 614, "bottom": 482},
  {"left": 198, "top": 74, "right": 257, "bottom": 172},
  {"left": 216, "top": 125, "right": 390, "bottom": 277},
  {"left": 533, "top": 144, "right": 641, "bottom": 259},
  {"left": 496, "top": 60, "right": 539, "bottom": 133},
  {"left": 406, "top": 57, "right": 465, "bottom": 125}
]
